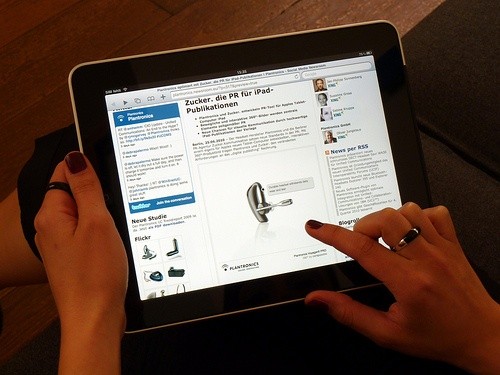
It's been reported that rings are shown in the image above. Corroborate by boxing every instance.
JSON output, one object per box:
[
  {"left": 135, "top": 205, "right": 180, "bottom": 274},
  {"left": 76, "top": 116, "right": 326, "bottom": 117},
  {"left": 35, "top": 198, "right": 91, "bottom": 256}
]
[
  {"left": 46, "top": 183, "right": 72, "bottom": 196},
  {"left": 392, "top": 227, "right": 420, "bottom": 252}
]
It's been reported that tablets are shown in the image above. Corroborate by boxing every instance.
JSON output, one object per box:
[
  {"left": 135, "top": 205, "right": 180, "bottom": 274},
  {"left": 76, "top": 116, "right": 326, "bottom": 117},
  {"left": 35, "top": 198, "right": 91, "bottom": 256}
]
[{"left": 68, "top": 21, "right": 433, "bottom": 333}]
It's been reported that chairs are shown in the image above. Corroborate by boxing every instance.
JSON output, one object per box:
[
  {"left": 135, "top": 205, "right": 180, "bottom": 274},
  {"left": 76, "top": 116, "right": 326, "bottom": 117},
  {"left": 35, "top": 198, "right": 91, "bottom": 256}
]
[{"left": 0, "top": 0, "right": 500, "bottom": 375}]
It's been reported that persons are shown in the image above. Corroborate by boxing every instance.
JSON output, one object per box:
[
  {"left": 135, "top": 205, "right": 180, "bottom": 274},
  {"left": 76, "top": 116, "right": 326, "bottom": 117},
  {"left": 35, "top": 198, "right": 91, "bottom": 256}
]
[
  {"left": 313, "top": 80, "right": 335, "bottom": 143},
  {"left": 0, "top": 150, "right": 500, "bottom": 375}
]
